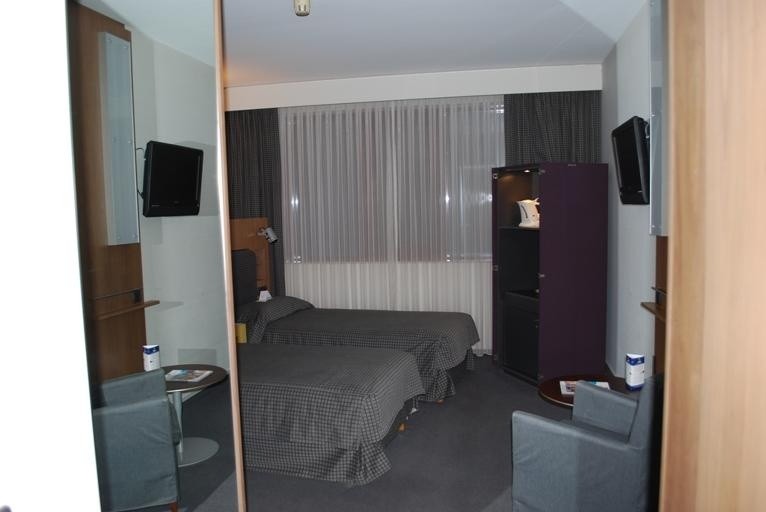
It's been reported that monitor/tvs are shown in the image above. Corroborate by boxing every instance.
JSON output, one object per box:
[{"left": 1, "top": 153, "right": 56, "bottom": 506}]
[
  {"left": 611, "top": 116, "right": 650, "bottom": 204},
  {"left": 142, "top": 140, "right": 204, "bottom": 218}
]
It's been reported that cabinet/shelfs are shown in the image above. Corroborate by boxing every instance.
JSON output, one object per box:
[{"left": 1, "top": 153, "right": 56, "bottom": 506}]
[{"left": 488, "top": 161, "right": 612, "bottom": 390}]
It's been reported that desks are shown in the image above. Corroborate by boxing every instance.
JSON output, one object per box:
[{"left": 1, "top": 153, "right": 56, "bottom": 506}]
[
  {"left": 151, "top": 363, "right": 227, "bottom": 472},
  {"left": 529, "top": 373, "right": 637, "bottom": 413}
]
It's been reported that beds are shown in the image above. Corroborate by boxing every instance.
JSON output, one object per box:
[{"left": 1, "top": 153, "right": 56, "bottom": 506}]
[
  {"left": 228, "top": 331, "right": 428, "bottom": 488},
  {"left": 229, "top": 241, "right": 481, "bottom": 405}
]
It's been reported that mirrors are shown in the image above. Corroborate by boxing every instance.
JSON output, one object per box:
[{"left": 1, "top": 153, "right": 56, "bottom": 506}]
[{"left": 66, "top": 0, "right": 250, "bottom": 511}]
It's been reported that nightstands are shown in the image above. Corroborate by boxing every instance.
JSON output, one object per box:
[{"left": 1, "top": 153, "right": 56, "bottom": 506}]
[{"left": 235, "top": 322, "right": 247, "bottom": 343}]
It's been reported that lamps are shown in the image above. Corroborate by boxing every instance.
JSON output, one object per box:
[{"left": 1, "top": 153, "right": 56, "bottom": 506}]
[{"left": 257, "top": 223, "right": 277, "bottom": 244}]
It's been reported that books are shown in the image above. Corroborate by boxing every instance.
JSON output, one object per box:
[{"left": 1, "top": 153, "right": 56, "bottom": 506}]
[
  {"left": 559, "top": 380, "right": 609, "bottom": 395},
  {"left": 165, "top": 368, "right": 213, "bottom": 382}
]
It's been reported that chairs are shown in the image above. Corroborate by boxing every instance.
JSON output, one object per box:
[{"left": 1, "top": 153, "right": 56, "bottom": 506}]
[
  {"left": 507, "top": 374, "right": 656, "bottom": 511},
  {"left": 87, "top": 370, "right": 184, "bottom": 511}
]
